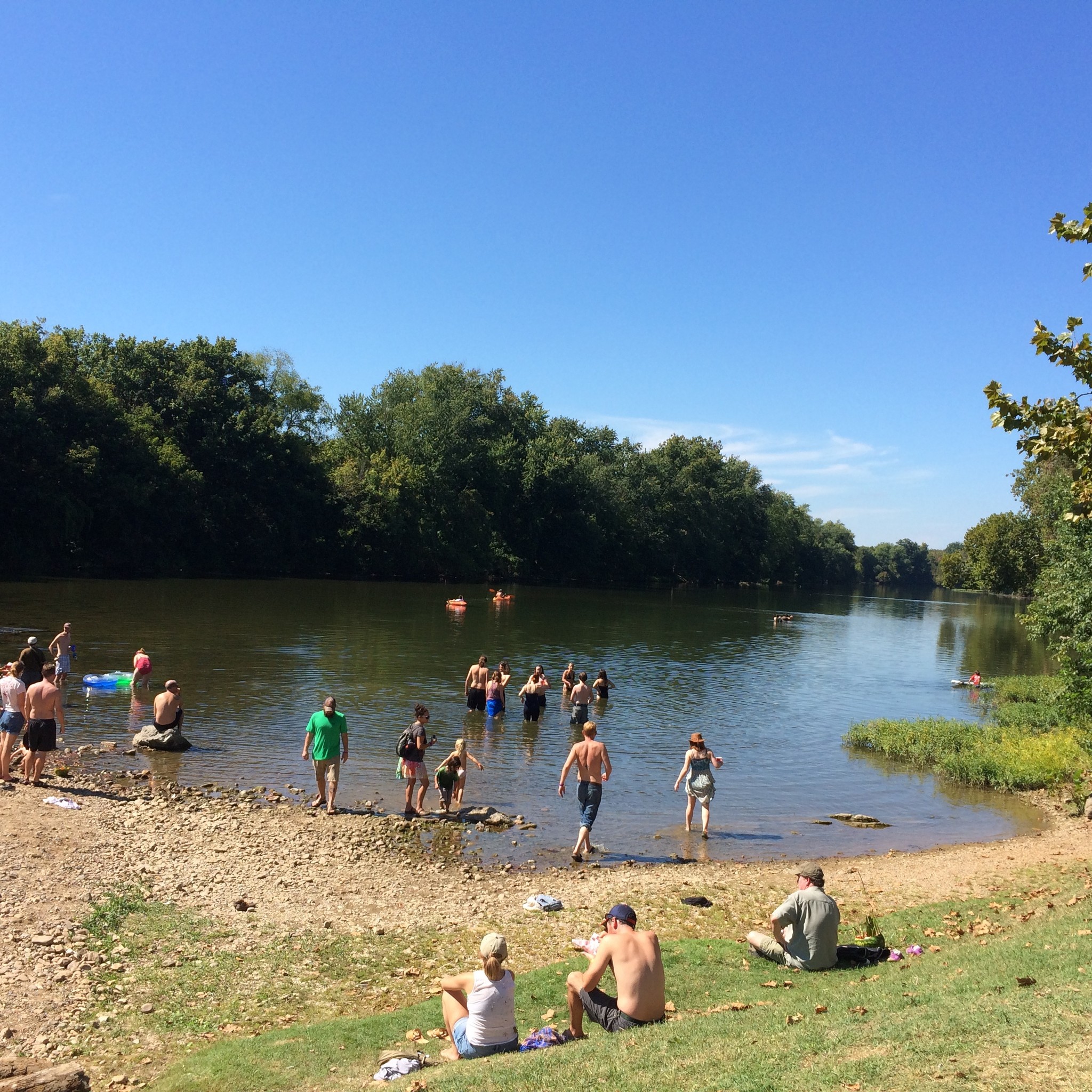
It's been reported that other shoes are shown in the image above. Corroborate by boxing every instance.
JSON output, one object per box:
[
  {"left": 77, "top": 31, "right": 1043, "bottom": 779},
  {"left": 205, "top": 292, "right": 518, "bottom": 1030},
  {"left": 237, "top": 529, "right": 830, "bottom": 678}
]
[{"left": 702, "top": 833, "right": 709, "bottom": 840}]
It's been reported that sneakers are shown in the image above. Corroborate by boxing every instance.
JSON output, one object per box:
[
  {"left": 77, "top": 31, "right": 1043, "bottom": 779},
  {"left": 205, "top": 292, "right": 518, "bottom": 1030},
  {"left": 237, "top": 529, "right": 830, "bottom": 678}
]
[
  {"left": 906, "top": 945, "right": 925, "bottom": 956},
  {"left": 887, "top": 949, "right": 903, "bottom": 962}
]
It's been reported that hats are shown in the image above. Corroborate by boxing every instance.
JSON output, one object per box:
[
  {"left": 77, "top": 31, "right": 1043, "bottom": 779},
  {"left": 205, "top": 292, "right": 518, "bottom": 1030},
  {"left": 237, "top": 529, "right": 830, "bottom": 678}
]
[
  {"left": 481, "top": 932, "right": 507, "bottom": 961},
  {"left": 601, "top": 904, "right": 637, "bottom": 928},
  {"left": 689, "top": 732, "right": 707, "bottom": 742},
  {"left": 324, "top": 696, "right": 336, "bottom": 712},
  {"left": 64, "top": 623, "right": 72, "bottom": 628},
  {"left": 794, "top": 862, "right": 824, "bottom": 879},
  {"left": 28, "top": 637, "right": 38, "bottom": 644}
]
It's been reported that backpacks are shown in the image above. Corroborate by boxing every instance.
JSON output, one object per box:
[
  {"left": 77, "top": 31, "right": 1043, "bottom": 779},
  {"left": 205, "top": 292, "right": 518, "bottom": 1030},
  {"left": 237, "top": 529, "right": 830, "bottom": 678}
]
[
  {"left": 396, "top": 724, "right": 423, "bottom": 758},
  {"left": 837, "top": 943, "right": 890, "bottom": 965},
  {"left": 537, "top": 894, "right": 563, "bottom": 912}
]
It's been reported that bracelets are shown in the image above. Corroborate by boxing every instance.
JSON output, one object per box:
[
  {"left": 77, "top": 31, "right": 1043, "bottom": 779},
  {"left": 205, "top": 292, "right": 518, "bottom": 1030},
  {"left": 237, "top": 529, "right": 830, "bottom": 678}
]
[
  {"left": 560, "top": 783, "right": 564, "bottom": 786},
  {"left": 429, "top": 742, "right": 432, "bottom": 747},
  {"left": 343, "top": 752, "right": 348, "bottom": 753},
  {"left": 719, "top": 761, "right": 722, "bottom": 765}
]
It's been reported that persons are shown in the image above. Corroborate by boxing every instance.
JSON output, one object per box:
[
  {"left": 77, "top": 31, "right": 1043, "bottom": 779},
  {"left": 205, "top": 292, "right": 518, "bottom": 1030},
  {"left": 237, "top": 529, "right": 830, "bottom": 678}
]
[
  {"left": 747, "top": 863, "right": 840, "bottom": 970},
  {"left": 674, "top": 732, "right": 723, "bottom": 839},
  {"left": 558, "top": 721, "right": 612, "bottom": 858},
  {"left": 0, "top": 622, "right": 75, "bottom": 786},
  {"left": 435, "top": 738, "right": 484, "bottom": 812},
  {"left": 969, "top": 670, "right": 981, "bottom": 688},
  {"left": 465, "top": 655, "right": 615, "bottom": 724},
  {"left": 774, "top": 613, "right": 792, "bottom": 622},
  {"left": 396, "top": 703, "right": 437, "bottom": 816},
  {"left": 152, "top": 680, "right": 185, "bottom": 733},
  {"left": 302, "top": 696, "right": 348, "bottom": 814},
  {"left": 130, "top": 648, "right": 153, "bottom": 688},
  {"left": 440, "top": 932, "right": 518, "bottom": 1061},
  {"left": 561, "top": 904, "right": 666, "bottom": 1042},
  {"left": 496, "top": 589, "right": 505, "bottom": 598},
  {"left": 450, "top": 595, "right": 465, "bottom": 601}
]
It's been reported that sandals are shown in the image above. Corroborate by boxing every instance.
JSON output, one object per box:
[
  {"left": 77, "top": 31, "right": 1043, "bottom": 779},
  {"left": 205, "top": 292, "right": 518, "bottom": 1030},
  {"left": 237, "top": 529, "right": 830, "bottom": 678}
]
[
  {"left": 311, "top": 795, "right": 335, "bottom": 815},
  {"left": 19, "top": 779, "right": 30, "bottom": 784},
  {"left": 31, "top": 780, "right": 50, "bottom": 788}
]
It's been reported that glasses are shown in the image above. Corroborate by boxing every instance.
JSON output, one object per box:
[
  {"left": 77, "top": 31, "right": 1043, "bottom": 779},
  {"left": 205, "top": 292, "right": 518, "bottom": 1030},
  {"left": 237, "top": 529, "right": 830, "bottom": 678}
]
[
  {"left": 420, "top": 715, "right": 429, "bottom": 719},
  {"left": 605, "top": 913, "right": 613, "bottom": 920},
  {"left": 173, "top": 685, "right": 178, "bottom": 688}
]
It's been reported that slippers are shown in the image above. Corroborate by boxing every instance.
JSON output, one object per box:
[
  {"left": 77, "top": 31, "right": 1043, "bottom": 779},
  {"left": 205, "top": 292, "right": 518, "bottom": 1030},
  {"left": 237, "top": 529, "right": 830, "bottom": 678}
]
[
  {"left": 571, "top": 853, "right": 583, "bottom": 862},
  {"left": 427, "top": 1028, "right": 451, "bottom": 1041},
  {"left": 405, "top": 1028, "right": 428, "bottom": 1044},
  {"left": 404, "top": 807, "right": 430, "bottom": 816},
  {"left": 561, "top": 1028, "right": 588, "bottom": 1041},
  {"left": 584, "top": 845, "right": 596, "bottom": 854},
  {"left": 5, "top": 777, "right": 19, "bottom": 782}
]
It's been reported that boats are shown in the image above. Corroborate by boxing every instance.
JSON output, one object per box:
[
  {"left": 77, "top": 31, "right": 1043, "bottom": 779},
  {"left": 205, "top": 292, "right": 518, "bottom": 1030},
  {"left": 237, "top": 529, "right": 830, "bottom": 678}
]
[
  {"left": 493, "top": 595, "right": 510, "bottom": 601},
  {"left": 83, "top": 671, "right": 138, "bottom": 687},
  {"left": 951, "top": 680, "right": 995, "bottom": 686},
  {"left": 0, "top": 662, "right": 13, "bottom": 674},
  {"left": 446, "top": 600, "right": 467, "bottom": 606}
]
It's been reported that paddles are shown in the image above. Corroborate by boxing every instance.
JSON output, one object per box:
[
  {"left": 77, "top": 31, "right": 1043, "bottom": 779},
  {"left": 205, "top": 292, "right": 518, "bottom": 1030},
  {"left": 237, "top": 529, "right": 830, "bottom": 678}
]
[{"left": 489, "top": 588, "right": 506, "bottom": 596}]
